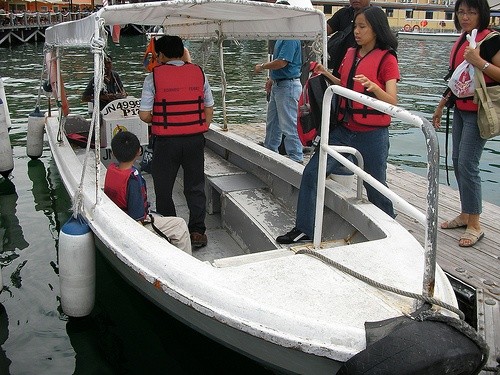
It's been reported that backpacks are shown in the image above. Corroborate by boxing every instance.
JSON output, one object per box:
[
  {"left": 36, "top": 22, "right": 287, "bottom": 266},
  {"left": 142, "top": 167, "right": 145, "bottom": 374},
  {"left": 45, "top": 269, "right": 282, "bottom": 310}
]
[{"left": 297, "top": 59, "right": 340, "bottom": 148}]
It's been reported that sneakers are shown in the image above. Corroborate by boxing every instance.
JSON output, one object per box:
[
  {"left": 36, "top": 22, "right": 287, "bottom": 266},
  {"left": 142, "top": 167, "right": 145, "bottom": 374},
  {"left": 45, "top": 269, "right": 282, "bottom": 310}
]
[
  {"left": 191, "top": 232, "right": 207, "bottom": 248},
  {"left": 275, "top": 227, "right": 313, "bottom": 244}
]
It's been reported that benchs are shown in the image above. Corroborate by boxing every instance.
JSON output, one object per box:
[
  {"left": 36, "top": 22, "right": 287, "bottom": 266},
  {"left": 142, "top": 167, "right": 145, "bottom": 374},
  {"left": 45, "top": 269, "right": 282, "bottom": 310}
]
[
  {"left": 75, "top": 144, "right": 108, "bottom": 194},
  {"left": 204, "top": 147, "right": 317, "bottom": 248}
]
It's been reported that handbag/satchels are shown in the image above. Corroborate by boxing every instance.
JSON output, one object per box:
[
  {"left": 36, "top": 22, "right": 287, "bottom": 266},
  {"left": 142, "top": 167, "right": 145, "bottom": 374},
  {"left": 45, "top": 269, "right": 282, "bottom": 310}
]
[
  {"left": 472, "top": 85, "right": 500, "bottom": 139},
  {"left": 448, "top": 29, "right": 481, "bottom": 98},
  {"left": 327, "top": 31, "right": 348, "bottom": 62}
]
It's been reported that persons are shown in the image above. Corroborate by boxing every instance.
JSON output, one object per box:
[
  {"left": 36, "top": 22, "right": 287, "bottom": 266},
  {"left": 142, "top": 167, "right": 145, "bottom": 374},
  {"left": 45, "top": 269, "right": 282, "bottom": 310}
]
[
  {"left": 138, "top": 35, "right": 214, "bottom": 248},
  {"left": 254, "top": 40, "right": 307, "bottom": 166},
  {"left": 103, "top": 132, "right": 193, "bottom": 257},
  {"left": 82, "top": 55, "right": 128, "bottom": 119},
  {"left": 322, "top": 0, "right": 378, "bottom": 74},
  {"left": 274, "top": 8, "right": 399, "bottom": 245},
  {"left": 433, "top": 0, "right": 500, "bottom": 247}
]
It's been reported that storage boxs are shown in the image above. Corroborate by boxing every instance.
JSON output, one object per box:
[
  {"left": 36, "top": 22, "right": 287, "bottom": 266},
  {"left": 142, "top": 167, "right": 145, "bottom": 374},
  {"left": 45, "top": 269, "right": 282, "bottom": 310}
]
[{"left": 99, "top": 117, "right": 149, "bottom": 152}]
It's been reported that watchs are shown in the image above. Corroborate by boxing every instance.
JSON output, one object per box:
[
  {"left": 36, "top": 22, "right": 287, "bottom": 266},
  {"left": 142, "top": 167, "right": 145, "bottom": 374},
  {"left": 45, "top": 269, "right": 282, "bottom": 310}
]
[{"left": 480, "top": 61, "right": 489, "bottom": 72}]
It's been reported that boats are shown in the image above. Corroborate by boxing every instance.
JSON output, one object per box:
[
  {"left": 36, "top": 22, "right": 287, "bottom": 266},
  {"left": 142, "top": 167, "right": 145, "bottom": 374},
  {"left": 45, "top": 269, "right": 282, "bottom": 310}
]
[
  {"left": 26, "top": 0, "right": 491, "bottom": 375},
  {"left": 397, "top": 19, "right": 463, "bottom": 42}
]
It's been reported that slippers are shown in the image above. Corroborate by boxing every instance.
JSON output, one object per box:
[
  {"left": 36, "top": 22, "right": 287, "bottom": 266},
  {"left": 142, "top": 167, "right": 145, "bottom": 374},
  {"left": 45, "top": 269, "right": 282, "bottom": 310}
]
[{"left": 441, "top": 216, "right": 484, "bottom": 247}]
